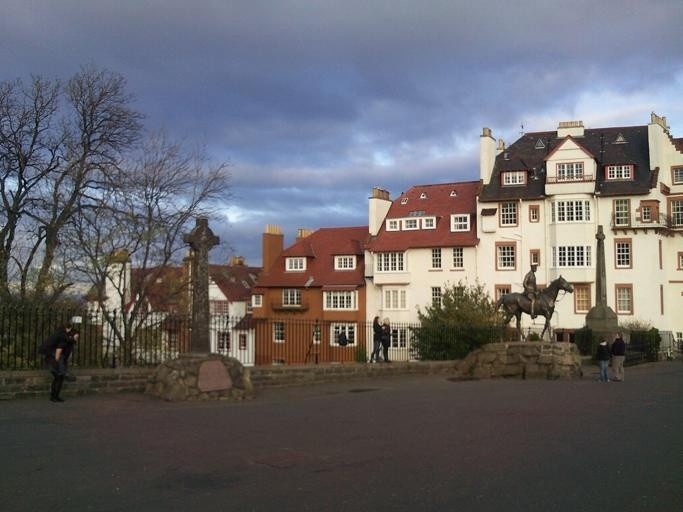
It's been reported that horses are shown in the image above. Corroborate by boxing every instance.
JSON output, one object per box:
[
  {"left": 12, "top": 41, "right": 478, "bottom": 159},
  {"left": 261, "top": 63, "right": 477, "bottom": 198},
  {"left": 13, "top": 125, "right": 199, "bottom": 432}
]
[{"left": 494, "top": 275, "right": 574, "bottom": 342}]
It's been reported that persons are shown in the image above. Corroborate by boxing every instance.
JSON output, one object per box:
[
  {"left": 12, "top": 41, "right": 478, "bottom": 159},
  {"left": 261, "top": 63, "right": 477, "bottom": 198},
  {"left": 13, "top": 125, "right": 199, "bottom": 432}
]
[
  {"left": 380, "top": 318, "right": 392, "bottom": 362},
  {"left": 596, "top": 338, "right": 611, "bottom": 384},
  {"left": 44, "top": 328, "right": 79, "bottom": 403},
  {"left": 39, "top": 324, "right": 77, "bottom": 382},
  {"left": 370, "top": 316, "right": 385, "bottom": 363},
  {"left": 523, "top": 263, "right": 540, "bottom": 319},
  {"left": 610, "top": 332, "right": 626, "bottom": 381}
]
